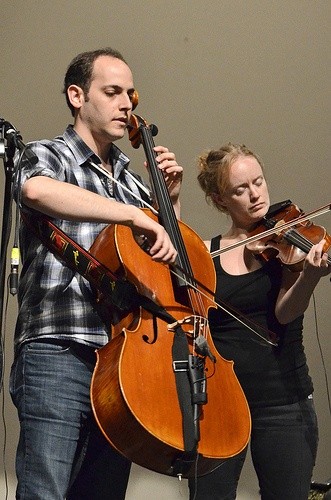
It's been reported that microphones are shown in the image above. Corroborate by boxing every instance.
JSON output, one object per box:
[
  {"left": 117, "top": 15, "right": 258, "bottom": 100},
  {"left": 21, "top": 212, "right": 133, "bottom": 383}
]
[{"left": 9, "top": 243, "right": 20, "bottom": 296}]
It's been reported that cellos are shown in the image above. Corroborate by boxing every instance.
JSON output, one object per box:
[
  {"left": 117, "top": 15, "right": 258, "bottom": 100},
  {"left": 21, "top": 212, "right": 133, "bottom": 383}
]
[{"left": 88, "top": 91, "right": 252, "bottom": 479}]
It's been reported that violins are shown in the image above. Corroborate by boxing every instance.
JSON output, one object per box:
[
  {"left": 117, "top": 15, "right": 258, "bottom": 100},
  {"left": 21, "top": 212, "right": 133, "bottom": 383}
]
[{"left": 244, "top": 200, "right": 330, "bottom": 279}]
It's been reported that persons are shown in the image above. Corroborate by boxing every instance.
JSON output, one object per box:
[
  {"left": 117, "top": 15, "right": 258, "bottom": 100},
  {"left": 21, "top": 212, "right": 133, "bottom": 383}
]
[
  {"left": 189, "top": 143, "right": 331, "bottom": 500},
  {"left": 9, "top": 48, "right": 184, "bottom": 500}
]
